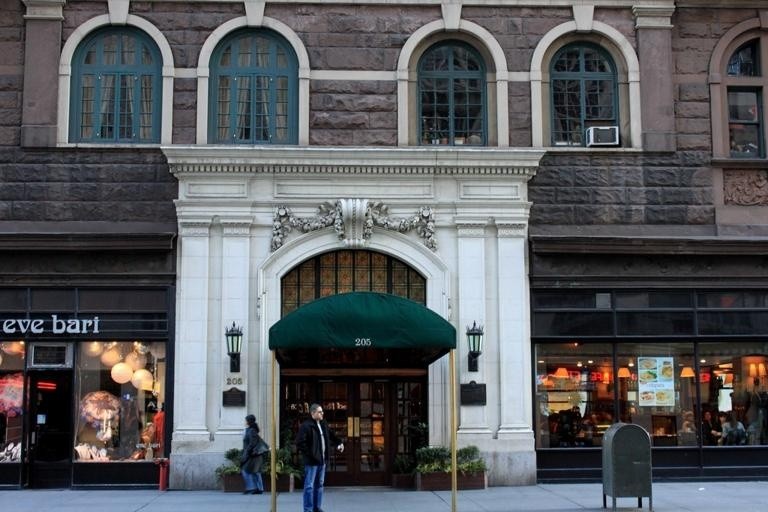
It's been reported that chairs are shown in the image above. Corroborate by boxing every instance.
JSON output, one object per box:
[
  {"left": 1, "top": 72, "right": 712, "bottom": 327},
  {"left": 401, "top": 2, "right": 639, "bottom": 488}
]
[{"left": 547, "top": 412, "right": 767, "bottom": 446}]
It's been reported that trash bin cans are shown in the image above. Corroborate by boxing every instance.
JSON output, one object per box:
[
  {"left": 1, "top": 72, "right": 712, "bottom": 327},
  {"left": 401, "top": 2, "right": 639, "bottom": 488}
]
[{"left": 603, "top": 422, "right": 652, "bottom": 511}]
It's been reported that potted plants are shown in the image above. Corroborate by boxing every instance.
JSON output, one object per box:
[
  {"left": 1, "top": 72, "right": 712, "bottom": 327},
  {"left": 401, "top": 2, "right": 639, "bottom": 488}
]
[
  {"left": 422, "top": 129, "right": 465, "bottom": 145},
  {"left": 391, "top": 450, "right": 416, "bottom": 488},
  {"left": 415, "top": 443, "right": 489, "bottom": 491},
  {"left": 215, "top": 447, "right": 304, "bottom": 493}
]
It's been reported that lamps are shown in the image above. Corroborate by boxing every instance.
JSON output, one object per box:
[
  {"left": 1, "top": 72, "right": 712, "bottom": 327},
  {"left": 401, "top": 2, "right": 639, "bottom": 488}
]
[
  {"left": 749, "top": 363, "right": 767, "bottom": 386},
  {"left": 617, "top": 355, "right": 630, "bottom": 377},
  {"left": 224, "top": 321, "right": 244, "bottom": 372},
  {"left": 553, "top": 344, "right": 569, "bottom": 377},
  {"left": 679, "top": 367, "right": 695, "bottom": 378},
  {"left": 465, "top": 320, "right": 485, "bottom": 371}
]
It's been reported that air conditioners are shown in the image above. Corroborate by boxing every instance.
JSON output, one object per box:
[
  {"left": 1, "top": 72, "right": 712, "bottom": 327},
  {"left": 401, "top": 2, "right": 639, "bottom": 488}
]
[{"left": 585, "top": 126, "right": 619, "bottom": 147}]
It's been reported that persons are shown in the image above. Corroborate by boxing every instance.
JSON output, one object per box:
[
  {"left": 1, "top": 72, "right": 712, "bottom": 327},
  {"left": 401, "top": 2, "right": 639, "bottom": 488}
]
[
  {"left": 544, "top": 405, "right": 594, "bottom": 447},
  {"left": 240, "top": 415, "right": 265, "bottom": 494},
  {"left": 683, "top": 411, "right": 745, "bottom": 446},
  {"left": 296, "top": 404, "right": 344, "bottom": 512}
]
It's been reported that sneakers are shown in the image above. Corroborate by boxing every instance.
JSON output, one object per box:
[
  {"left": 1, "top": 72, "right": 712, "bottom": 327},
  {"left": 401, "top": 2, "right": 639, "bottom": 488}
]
[{"left": 244, "top": 488, "right": 263, "bottom": 494}]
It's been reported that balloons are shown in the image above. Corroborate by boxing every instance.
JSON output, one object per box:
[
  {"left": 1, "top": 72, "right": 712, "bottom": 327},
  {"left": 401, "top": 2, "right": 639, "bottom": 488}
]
[
  {"left": 82, "top": 341, "right": 103, "bottom": 357},
  {"left": 125, "top": 351, "right": 148, "bottom": 370},
  {"left": 0, "top": 341, "right": 24, "bottom": 356},
  {"left": 111, "top": 363, "right": 133, "bottom": 384},
  {"left": 101, "top": 349, "right": 121, "bottom": 367},
  {"left": 131, "top": 369, "right": 154, "bottom": 391}
]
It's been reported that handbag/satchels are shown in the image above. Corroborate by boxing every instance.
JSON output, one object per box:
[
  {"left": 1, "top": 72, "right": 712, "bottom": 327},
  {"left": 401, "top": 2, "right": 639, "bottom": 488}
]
[{"left": 250, "top": 439, "right": 269, "bottom": 457}]
